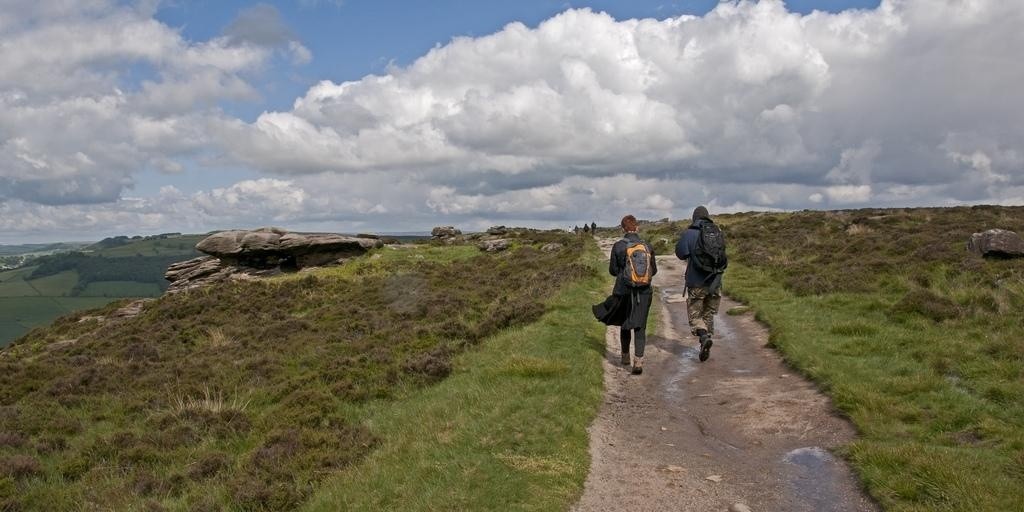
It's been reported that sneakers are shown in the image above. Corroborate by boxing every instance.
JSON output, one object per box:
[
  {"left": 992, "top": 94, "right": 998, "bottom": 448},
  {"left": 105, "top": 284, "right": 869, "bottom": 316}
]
[
  {"left": 621, "top": 352, "right": 631, "bottom": 364},
  {"left": 698, "top": 333, "right": 713, "bottom": 362},
  {"left": 631, "top": 359, "right": 643, "bottom": 375}
]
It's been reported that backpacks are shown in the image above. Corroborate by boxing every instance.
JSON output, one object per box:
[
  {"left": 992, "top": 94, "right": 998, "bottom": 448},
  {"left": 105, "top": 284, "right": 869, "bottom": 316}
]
[
  {"left": 688, "top": 220, "right": 728, "bottom": 274},
  {"left": 619, "top": 236, "right": 653, "bottom": 288}
]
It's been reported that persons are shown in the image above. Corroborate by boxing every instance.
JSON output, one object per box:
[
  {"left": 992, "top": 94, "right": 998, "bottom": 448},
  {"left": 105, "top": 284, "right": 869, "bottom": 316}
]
[
  {"left": 674, "top": 204, "right": 727, "bottom": 362},
  {"left": 567, "top": 225, "right": 572, "bottom": 235},
  {"left": 590, "top": 221, "right": 597, "bottom": 238},
  {"left": 574, "top": 224, "right": 578, "bottom": 234},
  {"left": 592, "top": 213, "right": 657, "bottom": 376},
  {"left": 583, "top": 223, "right": 589, "bottom": 233}
]
